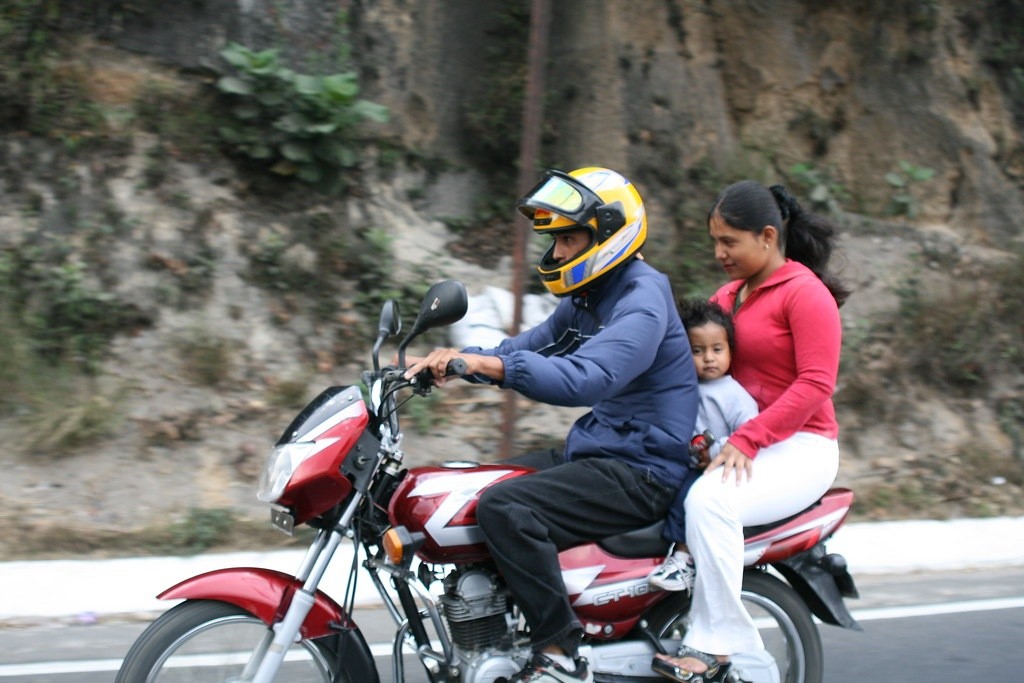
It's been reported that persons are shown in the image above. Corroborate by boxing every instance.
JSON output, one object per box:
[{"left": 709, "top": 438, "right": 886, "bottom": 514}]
[
  {"left": 389, "top": 168, "right": 697, "bottom": 682},
  {"left": 645, "top": 297, "right": 758, "bottom": 590},
  {"left": 652, "top": 180, "right": 852, "bottom": 683}
]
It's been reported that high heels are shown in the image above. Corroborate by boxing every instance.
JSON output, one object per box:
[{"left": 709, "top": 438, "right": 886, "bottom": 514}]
[{"left": 652, "top": 645, "right": 731, "bottom": 683}]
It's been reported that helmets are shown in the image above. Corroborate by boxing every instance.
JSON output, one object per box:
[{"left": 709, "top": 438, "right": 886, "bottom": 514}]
[{"left": 514, "top": 165, "right": 649, "bottom": 297}]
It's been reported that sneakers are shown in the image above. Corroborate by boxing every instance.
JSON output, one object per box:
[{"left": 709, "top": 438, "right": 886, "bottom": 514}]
[
  {"left": 505, "top": 652, "right": 593, "bottom": 683},
  {"left": 644, "top": 543, "right": 696, "bottom": 598}
]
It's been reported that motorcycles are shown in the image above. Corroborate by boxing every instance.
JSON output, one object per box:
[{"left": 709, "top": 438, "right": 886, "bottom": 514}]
[{"left": 113, "top": 278, "right": 864, "bottom": 682}]
